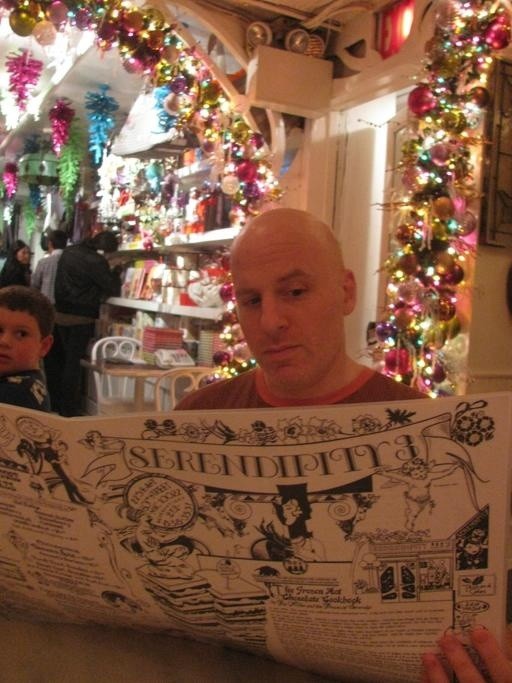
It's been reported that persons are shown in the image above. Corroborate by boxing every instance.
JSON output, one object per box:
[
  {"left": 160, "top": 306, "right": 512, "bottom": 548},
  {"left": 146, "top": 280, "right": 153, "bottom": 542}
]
[
  {"left": 0, "top": 228, "right": 126, "bottom": 412},
  {"left": 375, "top": 452, "right": 462, "bottom": 534},
  {"left": 44, "top": 430, "right": 121, "bottom": 504},
  {"left": 173, "top": 208, "right": 512, "bottom": 683}
]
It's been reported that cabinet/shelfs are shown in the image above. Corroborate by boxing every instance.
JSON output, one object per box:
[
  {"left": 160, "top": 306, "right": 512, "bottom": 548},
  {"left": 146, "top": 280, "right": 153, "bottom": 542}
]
[{"left": 98, "top": 140, "right": 289, "bottom": 321}]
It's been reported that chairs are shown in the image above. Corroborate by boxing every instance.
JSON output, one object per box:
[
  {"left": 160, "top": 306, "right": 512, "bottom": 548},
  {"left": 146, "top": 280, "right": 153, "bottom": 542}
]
[{"left": 79, "top": 334, "right": 222, "bottom": 417}]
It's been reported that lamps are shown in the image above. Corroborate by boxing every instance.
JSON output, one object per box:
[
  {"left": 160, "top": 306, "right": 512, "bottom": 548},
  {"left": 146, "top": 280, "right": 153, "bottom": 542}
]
[{"left": 240, "top": 17, "right": 329, "bottom": 65}]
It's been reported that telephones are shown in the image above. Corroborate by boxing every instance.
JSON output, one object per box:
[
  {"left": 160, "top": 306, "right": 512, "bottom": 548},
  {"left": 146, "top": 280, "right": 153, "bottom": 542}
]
[{"left": 153, "top": 348, "right": 196, "bottom": 369}]
